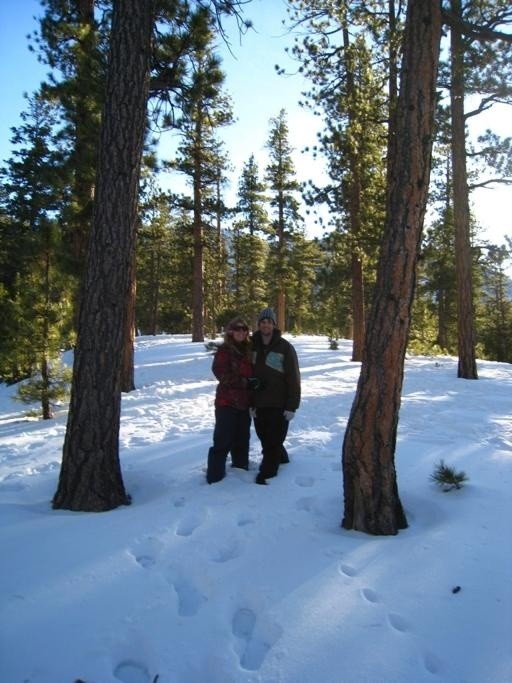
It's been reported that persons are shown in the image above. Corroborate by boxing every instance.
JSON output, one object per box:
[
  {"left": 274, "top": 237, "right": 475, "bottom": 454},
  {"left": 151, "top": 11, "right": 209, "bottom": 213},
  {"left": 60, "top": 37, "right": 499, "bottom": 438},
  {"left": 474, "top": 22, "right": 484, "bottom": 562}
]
[
  {"left": 205, "top": 316, "right": 260, "bottom": 484},
  {"left": 248, "top": 307, "right": 301, "bottom": 485}
]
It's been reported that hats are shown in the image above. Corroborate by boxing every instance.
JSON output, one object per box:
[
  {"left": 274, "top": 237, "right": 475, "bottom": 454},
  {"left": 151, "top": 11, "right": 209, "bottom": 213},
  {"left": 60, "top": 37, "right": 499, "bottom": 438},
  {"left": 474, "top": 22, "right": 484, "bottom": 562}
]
[{"left": 258, "top": 307, "right": 276, "bottom": 325}]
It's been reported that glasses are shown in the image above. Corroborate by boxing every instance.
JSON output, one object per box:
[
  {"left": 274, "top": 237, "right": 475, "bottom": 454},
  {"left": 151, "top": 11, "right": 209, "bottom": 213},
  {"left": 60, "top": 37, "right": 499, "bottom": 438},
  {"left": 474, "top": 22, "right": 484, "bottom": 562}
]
[{"left": 229, "top": 325, "right": 248, "bottom": 331}]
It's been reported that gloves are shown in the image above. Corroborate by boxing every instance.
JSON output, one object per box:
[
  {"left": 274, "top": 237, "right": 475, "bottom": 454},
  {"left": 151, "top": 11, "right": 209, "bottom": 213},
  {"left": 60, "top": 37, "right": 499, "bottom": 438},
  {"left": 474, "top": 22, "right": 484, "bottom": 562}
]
[
  {"left": 242, "top": 376, "right": 260, "bottom": 390},
  {"left": 283, "top": 410, "right": 295, "bottom": 420},
  {"left": 249, "top": 407, "right": 256, "bottom": 417}
]
